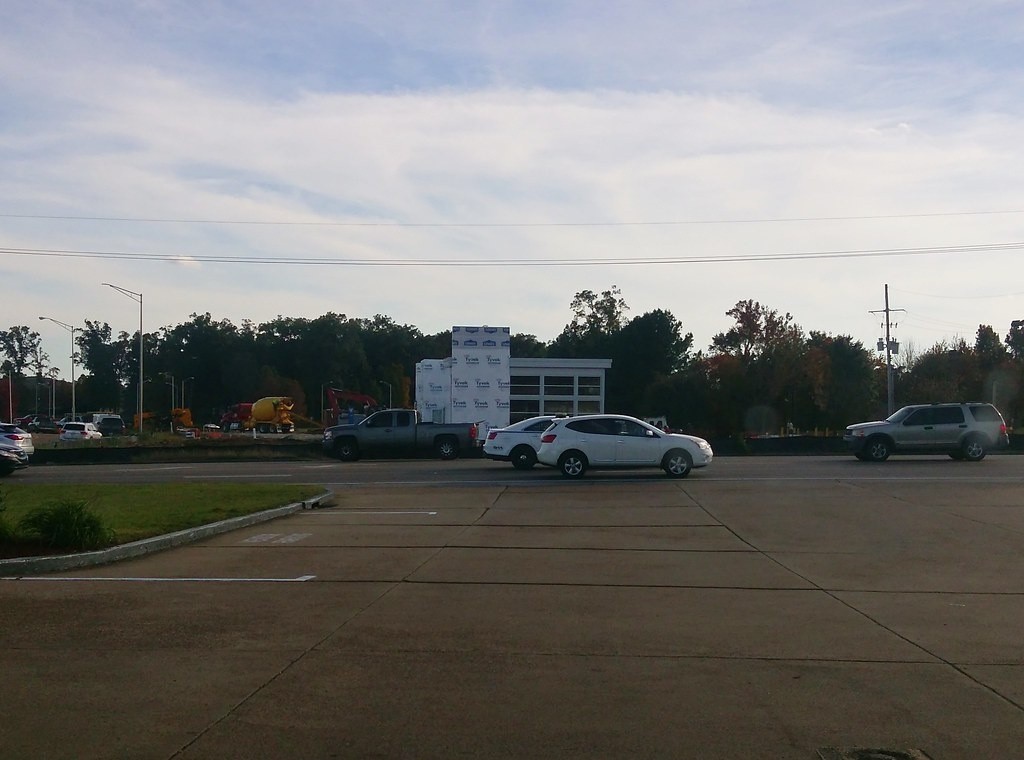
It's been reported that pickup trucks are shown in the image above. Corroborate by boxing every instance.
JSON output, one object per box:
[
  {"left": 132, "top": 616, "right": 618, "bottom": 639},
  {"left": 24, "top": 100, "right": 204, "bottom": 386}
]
[
  {"left": 843, "top": 402, "right": 1010, "bottom": 462},
  {"left": 322, "top": 408, "right": 479, "bottom": 462}
]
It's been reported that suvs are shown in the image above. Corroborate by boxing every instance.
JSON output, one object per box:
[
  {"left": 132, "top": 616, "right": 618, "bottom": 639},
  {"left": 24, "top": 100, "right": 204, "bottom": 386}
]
[
  {"left": 0, "top": 422, "right": 35, "bottom": 456},
  {"left": 59, "top": 422, "right": 102, "bottom": 442},
  {"left": 53, "top": 415, "right": 84, "bottom": 428},
  {"left": 13, "top": 414, "right": 48, "bottom": 425}
]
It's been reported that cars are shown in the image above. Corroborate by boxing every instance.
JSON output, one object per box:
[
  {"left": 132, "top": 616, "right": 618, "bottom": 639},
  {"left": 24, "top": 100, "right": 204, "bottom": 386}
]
[
  {"left": 483, "top": 416, "right": 571, "bottom": 469},
  {"left": 0, "top": 444, "right": 29, "bottom": 478},
  {"left": 28, "top": 416, "right": 41, "bottom": 425},
  {"left": 536, "top": 413, "right": 713, "bottom": 480}
]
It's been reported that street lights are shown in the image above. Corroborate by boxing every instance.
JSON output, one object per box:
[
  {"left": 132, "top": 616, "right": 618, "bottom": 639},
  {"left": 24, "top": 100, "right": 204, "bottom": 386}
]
[
  {"left": 39, "top": 316, "right": 76, "bottom": 421},
  {"left": 102, "top": 282, "right": 143, "bottom": 432},
  {"left": 321, "top": 380, "right": 333, "bottom": 423},
  {"left": 379, "top": 381, "right": 391, "bottom": 408},
  {"left": 159, "top": 372, "right": 174, "bottom": 410},
  {"left": 182, "top": 377, "right": 195, "bottom": 410}
]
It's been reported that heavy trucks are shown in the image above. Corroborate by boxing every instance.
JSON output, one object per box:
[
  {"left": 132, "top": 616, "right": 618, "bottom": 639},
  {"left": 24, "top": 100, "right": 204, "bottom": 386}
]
[{"left": 219, "top": 402, "right": 295, "bottom": 434}]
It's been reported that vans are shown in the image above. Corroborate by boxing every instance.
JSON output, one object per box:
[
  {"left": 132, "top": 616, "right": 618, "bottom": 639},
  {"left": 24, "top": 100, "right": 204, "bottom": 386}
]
[{"left": 203, "top": 424, "right": 219, "bottom": 432}]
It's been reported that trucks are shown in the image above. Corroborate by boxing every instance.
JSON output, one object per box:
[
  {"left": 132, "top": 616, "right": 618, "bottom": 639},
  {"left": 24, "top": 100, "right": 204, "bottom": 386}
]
[
  {"left": 92, "top": 414, "right": 126, "bottom": 435},
  {"left": 186, "top": 428, "right": 201, "bottom": 440},
  {"left": 339, "top": 413, "right": 366, "bottom": 425}
]
